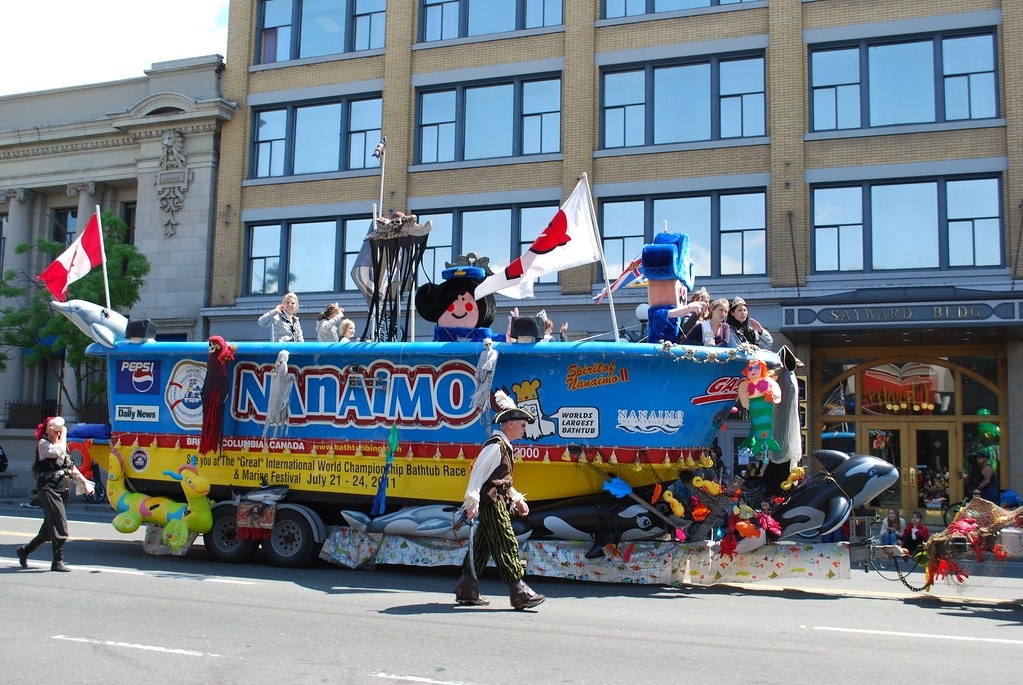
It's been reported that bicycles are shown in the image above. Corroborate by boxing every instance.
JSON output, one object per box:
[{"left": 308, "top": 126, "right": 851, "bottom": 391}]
[
  {"left": 944, "top": 488, "right": 1019, "bottom": 529},
  {"left": 83, "top": 460, "right": 106, "bottom": 503}
]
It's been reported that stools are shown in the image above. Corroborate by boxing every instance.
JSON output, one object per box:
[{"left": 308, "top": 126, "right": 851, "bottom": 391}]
[{"left": 0, "top": 472, "right": 18, "bottom": 497}]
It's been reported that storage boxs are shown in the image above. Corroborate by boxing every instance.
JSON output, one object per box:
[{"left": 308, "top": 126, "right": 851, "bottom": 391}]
[{"left": 1000, "top": 526, "right": 1023, "bottom": 557}]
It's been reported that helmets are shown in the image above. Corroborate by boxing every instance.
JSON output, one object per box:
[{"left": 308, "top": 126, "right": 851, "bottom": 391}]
[{"left": 977, "top": 451, "right": 990, "bottom": 458}]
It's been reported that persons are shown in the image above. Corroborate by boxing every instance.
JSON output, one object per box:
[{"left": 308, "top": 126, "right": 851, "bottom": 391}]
[
  {"left": 969, "top": 487, "right": 983, "bottom": 500},
  {"left": 975, "top": 452, "right": 1001, "bottom": 506},
  {"left": 315, "top": 303, "right": 344, "bottom": 342},
  {"left": 337, "top": 319, "right": 356, "bottom": 342},
  {"left": 454, "top": 407, "right": 547, "bottom": 612},
  {"left": 257, "top": 292, "right": 306, "bottom": 342},
  {"left": 680, "top": 289, "right": 774, "bottom": 350},
  {"left": 879, "top": 506, "right": 906, "bottom": 545},
  {"left": 537, "top": 316, "right": 568, "bottom": 343},
  {"left": 903, "top": 510, "right": 929, "bottom": 555},
  {"left": 16, "top": 417, "right": 96, "bottom": 572}
]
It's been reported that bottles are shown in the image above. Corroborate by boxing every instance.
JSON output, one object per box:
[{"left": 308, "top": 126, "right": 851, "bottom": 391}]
[{"left": 53, "top": 416, "right": 65, "bottom": 431}]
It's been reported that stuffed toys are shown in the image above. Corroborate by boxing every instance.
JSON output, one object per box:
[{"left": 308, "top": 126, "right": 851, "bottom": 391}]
[{"left": 641, "top": 232, "right": 705, "bottom": 343}]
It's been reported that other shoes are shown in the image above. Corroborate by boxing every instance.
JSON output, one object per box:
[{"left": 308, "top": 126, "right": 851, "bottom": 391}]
[
  {"left": 16, "top": 545, "right": 28, "bottom": 568},
  {"left": 51, "top": 561, "right": 71, "bottom": 572}
]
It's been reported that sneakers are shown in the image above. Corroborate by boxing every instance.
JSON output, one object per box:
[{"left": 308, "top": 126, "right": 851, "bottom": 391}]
[
  {"left": 455, "top": 595, "right": 489, "bottom": 606},
  {"left": 514, "top": 594, "right": 545, "bottom": 610}
]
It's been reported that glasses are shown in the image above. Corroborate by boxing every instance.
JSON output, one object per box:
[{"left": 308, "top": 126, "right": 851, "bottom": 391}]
[{"left": 973, "top": 493, "right": 982, "bottom": 495}]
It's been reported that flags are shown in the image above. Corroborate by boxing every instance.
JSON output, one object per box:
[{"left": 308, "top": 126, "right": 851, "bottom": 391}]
[
  {"left": 35, "top": 213, "right": 103, "bottom": 302},
  {"left": 473, "top": 173, "right": 602, "bottom": 300}
]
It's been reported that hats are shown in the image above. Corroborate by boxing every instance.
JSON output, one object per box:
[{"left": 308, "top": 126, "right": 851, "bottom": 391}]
[{"left": 492, "top": 390, "right": 536, "bottom": 425}]
